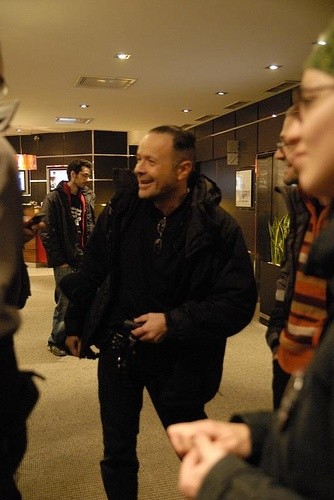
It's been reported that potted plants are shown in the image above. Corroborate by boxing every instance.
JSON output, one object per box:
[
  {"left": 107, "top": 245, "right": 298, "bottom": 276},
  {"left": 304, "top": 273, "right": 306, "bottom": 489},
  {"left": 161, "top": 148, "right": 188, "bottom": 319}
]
[{"left": 259, "top": 215, "right": 290, "bottom": 328}]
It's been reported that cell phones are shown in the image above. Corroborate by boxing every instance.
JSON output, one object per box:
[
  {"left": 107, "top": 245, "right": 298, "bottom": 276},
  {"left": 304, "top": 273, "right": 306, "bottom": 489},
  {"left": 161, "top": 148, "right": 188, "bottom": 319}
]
[{"left": 24, "top": 213, "right": 45, "bottom": 230}]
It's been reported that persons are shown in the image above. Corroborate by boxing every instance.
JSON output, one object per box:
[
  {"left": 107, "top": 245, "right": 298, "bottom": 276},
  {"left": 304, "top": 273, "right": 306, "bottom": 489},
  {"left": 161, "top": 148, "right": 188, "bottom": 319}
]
[
  {"left": 57, "top": 125, "right": 257, "bottom": 500},
  {"left": 166, "top": 24, "right": 334, "bottom": 500},
  {"left": 38, "top": 159, "right": 96, "bottom": 357},
  {"left": 0, "top": 49, "right": 32, "bottom": 500}
]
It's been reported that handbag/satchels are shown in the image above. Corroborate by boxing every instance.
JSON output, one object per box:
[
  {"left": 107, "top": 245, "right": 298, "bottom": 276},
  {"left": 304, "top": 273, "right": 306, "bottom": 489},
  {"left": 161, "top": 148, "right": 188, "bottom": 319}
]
[{"left": 52, "top": 265, "right": 115, "bottom": 354}]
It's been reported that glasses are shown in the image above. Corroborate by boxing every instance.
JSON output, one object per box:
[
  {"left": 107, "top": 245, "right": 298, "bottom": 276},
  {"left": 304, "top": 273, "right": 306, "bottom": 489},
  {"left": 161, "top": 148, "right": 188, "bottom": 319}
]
[
  {"left": 155, "top": 217, "right": 167, "bottom": 252},
  {"left": 293, "top": 83, "right": 334, "bottom": 115},
  {"left": 277, "top": 136, "right": 294, "bottom": 157}
]
